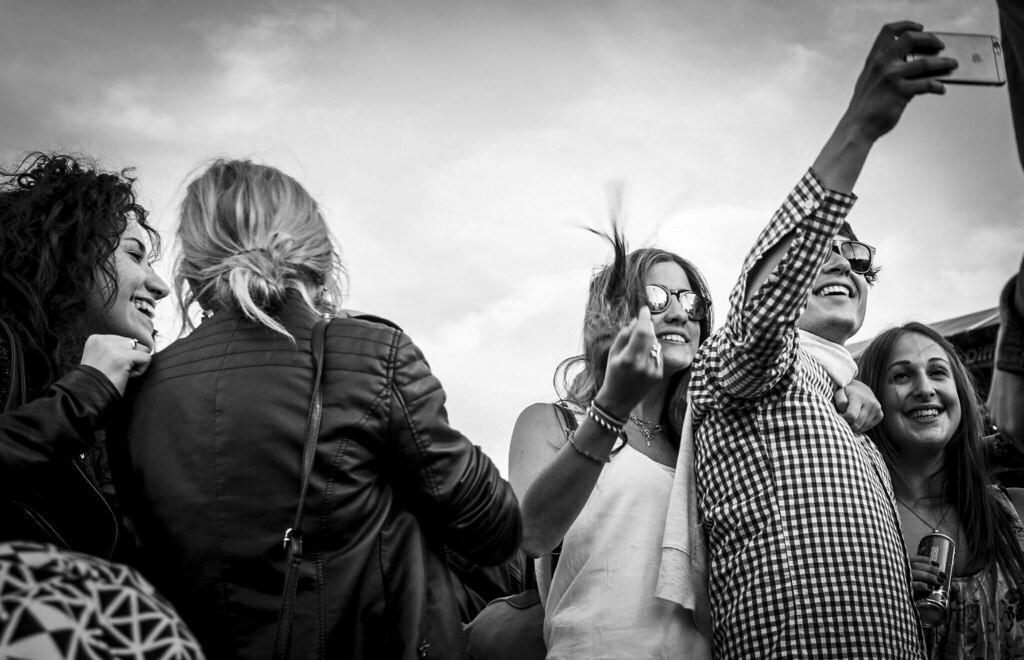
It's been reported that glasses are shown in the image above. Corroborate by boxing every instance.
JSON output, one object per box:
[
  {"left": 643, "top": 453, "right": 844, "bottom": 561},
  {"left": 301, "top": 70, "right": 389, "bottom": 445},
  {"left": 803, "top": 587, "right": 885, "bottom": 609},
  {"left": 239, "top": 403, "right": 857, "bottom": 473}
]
[
  {"left": 824, "top": 239, "right": 877, "bottom": 274},
  {"left": 645, "top": 285, "right": 713, "bottom": 322}
]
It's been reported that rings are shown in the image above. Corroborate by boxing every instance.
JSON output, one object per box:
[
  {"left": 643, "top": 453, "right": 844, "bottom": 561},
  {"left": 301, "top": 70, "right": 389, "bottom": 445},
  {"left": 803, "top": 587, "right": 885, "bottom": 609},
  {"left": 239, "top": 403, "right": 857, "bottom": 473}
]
[
  {"left": 129, "top": 338, "right": 139, "bottom": 350},
  {"left": 649, "top": 345, "right": 659, "bottom": 358}
]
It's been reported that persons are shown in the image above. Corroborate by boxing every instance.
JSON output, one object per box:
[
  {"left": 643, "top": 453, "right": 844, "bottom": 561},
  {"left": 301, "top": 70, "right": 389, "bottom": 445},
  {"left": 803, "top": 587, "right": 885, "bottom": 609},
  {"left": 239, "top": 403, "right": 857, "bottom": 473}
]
[
  {"left": 112, "top": 158, "right": 522, "bottom": 659},
  {"left": 508, "top": 231, "right": 725, "bottom": 660},
  {"left": 0, "top": 149, "right": 170, "bottom": 570},
  {"left": 689, "top": 20, "right": 934, "bottom": 660},
  {"left": 852, "top": 319, "right": 1024, "bottom": 660}
]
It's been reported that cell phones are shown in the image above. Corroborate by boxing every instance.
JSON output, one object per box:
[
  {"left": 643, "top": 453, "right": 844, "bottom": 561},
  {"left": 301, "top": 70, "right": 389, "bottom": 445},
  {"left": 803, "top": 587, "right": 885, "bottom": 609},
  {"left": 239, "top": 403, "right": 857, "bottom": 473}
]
[{"left": 907, "top": 32, "right": 1005, "bottom": 86}]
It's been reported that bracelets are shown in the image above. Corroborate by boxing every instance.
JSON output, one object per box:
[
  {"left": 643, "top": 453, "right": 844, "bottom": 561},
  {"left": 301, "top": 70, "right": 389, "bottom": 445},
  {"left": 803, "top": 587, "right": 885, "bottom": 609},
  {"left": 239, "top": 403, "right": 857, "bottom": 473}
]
[
  {"left": 584, "top": 406, "right": 625, "bottom": 435},
  {"left": 590, "top": 398, "right": 628, "bottom": 427},
  {"left": 566, "top": 431, "right": 610, "bottom": 465}
]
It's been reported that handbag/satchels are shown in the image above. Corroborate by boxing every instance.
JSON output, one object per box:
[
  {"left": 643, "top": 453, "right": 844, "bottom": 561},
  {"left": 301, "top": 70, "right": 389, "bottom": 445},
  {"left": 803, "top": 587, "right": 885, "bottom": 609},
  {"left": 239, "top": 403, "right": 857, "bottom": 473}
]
[{"left": 465, "top": 589, "right": 548, "bottom": 660}]
